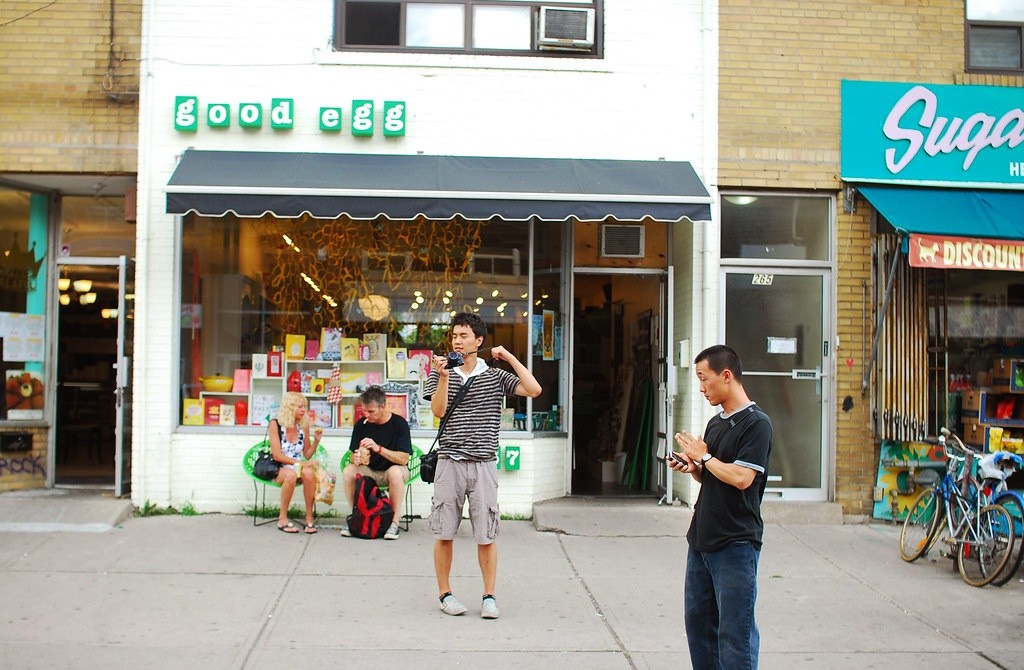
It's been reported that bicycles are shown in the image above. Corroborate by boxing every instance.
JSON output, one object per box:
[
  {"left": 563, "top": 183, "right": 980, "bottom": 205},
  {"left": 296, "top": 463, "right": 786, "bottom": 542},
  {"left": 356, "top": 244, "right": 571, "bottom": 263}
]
[{"left": 901, "top": 425, "right": 1023, "bottom": 588}]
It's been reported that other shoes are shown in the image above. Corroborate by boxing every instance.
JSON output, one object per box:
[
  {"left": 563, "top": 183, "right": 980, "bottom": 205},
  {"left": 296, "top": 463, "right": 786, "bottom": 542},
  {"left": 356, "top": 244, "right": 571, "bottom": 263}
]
[
  {"left": 439, "top": 596, "right": 468, "bottom": 616},
  {"left": 481, "top": 598, "right": 498, "bottom": 619}
]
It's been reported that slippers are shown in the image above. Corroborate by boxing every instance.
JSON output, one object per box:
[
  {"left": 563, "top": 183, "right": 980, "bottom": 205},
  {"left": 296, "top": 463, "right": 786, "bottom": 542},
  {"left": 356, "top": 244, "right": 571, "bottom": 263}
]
[
  {"left": 304, "top": 524, "right": 316, "bottom": 534},
  {"left": 278, "top": 521, "right": 299, "bottom": 533}
]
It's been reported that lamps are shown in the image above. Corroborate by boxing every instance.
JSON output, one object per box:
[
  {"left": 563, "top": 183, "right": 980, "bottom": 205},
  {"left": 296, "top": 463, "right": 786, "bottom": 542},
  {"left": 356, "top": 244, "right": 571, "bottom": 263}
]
[
  {"left": 357, "top": 294, "right": 391, "bottom": 320},
  {"left": 58, "top": 278, "right": 119, "bottom": 319}
]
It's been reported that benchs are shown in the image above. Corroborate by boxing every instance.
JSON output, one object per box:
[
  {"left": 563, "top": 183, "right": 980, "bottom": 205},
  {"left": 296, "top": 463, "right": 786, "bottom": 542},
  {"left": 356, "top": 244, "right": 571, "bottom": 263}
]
[{"left": 60, "top": 424, "right": 105, "bottom": 466}]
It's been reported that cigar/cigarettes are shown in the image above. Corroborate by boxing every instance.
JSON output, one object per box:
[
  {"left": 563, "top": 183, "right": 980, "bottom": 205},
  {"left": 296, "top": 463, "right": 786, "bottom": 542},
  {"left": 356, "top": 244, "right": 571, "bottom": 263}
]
[{"left": 363, "top": 417, "right": 369, "bottom": 424}]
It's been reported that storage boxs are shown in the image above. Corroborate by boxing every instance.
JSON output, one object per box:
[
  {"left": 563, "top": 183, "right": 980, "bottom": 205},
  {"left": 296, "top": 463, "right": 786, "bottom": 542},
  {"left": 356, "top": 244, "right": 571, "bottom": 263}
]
[
  {"left": 183, "top": 397, "right": 247, "bottom": 425},
  {"left": 234, "top": 327, "right": 442, "bottom": 427}
]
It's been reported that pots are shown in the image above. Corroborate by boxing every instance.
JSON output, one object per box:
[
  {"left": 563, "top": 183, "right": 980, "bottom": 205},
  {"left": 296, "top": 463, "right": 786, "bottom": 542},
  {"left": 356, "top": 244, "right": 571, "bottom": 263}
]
[{"left": 199, "top": 373, "right": 233, "bottom": 392}]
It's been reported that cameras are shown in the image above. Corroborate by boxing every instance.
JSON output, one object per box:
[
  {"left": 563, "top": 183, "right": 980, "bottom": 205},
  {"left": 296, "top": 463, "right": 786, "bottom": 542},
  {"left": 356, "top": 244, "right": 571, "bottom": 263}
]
[{"left": 442, "top": 351, "right": 464, "bottom": 369}]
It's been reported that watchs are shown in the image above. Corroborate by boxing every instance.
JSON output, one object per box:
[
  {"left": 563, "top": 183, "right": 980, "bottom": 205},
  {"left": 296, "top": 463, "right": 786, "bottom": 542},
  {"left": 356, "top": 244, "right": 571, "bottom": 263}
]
[{"left": 701, "top": 453, "right": 714, "bottom": 467}]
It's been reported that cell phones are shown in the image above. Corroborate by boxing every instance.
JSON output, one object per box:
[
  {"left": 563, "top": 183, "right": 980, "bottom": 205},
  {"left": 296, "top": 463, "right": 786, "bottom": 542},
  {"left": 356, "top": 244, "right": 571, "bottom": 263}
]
[{"left": 669, "top": 452, "right": 688, "bottom": 469}]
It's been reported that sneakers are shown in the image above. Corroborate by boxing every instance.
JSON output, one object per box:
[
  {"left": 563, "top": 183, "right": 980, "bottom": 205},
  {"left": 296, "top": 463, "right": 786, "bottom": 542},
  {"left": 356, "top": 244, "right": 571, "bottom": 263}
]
[
  {"left": 383, "top": 523, "right": 401, "bottom": 539},
  {"left": 340, "top": 528, "right": 351, "bottom": 537}
]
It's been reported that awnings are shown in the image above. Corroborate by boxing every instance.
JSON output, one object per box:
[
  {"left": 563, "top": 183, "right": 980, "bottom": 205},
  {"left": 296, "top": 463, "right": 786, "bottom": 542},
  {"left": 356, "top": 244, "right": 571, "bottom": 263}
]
[
  {"left": 163, "top": 149, "right": 712, "bottom": 223},
  {"left": 854, "top": 186, "right": 1024, "bottom": 239}
]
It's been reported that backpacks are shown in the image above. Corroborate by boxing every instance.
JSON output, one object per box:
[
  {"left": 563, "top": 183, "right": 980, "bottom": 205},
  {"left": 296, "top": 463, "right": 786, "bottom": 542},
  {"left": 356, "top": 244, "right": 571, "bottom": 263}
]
[{"left": 346, "top": 474, "right": 395, "bottom": 539}]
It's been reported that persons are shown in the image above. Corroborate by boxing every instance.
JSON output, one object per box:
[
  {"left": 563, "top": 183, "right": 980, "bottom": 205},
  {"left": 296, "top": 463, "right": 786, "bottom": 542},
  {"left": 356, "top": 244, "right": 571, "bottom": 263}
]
[
  {"left": 254, "top": 390, "right": 324, "bottom": 534},
  {"left": 423, "top": 312, "right": 542, "bottom": 618},
  {"left": 665, "top": 344, "right": 773, "bottom": 670},
  {"left": 341, "top": 385, "right": 413, "bottom": 540}
]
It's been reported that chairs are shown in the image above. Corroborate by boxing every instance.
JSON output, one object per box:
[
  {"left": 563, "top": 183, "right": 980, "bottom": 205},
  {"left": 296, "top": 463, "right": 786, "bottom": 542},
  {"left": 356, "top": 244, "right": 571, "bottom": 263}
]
[
  {"left": 243, "top": 439, "right": 329, "bottom": 527},
  {"left": 341, "top": 443, "right": 424, "bottom": 531}
]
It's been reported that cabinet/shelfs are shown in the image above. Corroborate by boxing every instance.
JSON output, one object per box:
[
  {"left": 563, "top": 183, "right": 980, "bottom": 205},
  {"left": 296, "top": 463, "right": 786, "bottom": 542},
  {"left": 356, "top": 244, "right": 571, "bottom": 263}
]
[
  {"left": 960, "top": 358, "right": 1024, "bottom": 457},
  {"left": 199, "top": 359, "right": 425, "bottom": 430},
  {"left": 203, "top": 273, "right": 277, "bottom": 379}
]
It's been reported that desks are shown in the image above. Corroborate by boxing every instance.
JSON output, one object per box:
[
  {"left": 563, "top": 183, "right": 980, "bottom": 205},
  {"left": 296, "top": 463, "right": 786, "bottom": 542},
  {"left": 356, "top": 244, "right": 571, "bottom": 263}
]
[{"left": 57, "top": 385, "right": 111, "bottom": 416}]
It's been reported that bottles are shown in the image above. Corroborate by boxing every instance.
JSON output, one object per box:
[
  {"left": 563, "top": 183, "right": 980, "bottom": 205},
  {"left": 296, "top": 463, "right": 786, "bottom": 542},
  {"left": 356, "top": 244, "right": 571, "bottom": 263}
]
[{"left": 358, "top": 443, "right": 370, "bottom": 465}]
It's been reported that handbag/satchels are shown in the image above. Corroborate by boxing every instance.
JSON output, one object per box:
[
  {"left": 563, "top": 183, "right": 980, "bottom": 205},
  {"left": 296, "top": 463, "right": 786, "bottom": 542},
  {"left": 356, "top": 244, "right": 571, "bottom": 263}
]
[
  {"left": 421, "top": 450, "right": 439, "bottom": 485},
  {"left": 254, "top": 419, "right": 282, "bottom": 480}
]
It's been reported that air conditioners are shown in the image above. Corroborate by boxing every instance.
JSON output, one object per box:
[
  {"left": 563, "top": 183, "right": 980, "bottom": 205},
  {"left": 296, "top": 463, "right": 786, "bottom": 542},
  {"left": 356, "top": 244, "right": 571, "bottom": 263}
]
[
  {"left": 600, "top": 223, "right": 646, "bottom": 258},
  {"left": 536, "top": 4, "right": 595, "bottom": 52}
]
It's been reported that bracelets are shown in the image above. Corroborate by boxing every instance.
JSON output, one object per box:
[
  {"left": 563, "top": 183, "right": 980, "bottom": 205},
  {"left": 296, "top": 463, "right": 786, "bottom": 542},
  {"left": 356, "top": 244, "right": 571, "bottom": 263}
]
[{"left": 377, "top": 445, "right": 382, "bottom": 455}]
